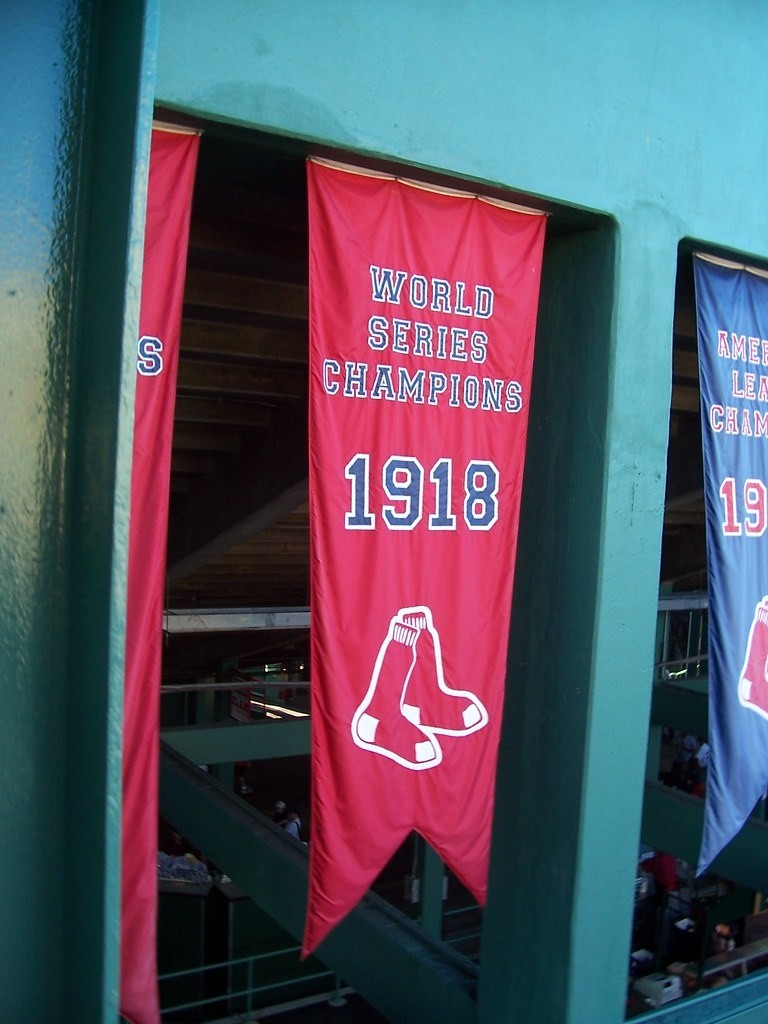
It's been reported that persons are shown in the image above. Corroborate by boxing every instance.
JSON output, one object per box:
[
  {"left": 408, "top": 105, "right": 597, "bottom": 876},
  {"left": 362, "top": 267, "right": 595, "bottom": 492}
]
[
  {"left": 663, "top": 727, "right": 709, "bottom": 798},
  {"left": 634, "top": 849, "right": 748, "bottom": 995},
  {"left": 156, "top": 763, "right": 308, "bottom": 869}
]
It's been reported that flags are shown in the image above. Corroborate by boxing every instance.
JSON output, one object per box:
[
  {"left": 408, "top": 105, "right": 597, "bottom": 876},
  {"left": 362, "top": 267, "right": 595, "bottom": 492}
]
[
  {"left": 692, "top": 247, "right": 768, "bottom": 876},
  {"left": 290, "top": 152, "right": 546, "bottom": 959},
  {"left": 116, "top": 116, "right": 201, "bottom": 1023}
]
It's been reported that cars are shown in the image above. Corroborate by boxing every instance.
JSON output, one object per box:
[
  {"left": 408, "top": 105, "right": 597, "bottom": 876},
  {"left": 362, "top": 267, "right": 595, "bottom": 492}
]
[{"left": 635, "top": 846, "right": 729, "bottom": 904}]
